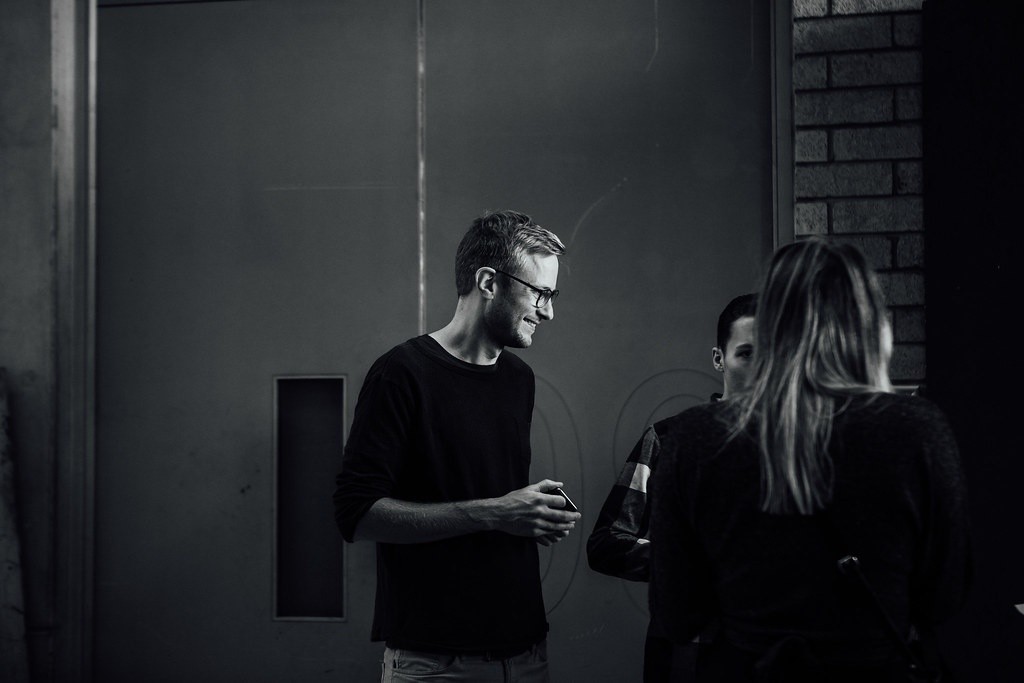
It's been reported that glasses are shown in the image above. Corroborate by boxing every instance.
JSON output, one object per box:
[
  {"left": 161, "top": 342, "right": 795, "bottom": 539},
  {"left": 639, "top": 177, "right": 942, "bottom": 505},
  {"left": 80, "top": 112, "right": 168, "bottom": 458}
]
[{"left": 495, "top": 269, "right": 560, "bottom": 308}]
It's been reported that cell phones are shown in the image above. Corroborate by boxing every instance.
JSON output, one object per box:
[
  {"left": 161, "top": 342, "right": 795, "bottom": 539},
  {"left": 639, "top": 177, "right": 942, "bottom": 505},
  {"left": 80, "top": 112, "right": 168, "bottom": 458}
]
[{"left": 546, "top": 487, "right": 578, "bottom": 512}]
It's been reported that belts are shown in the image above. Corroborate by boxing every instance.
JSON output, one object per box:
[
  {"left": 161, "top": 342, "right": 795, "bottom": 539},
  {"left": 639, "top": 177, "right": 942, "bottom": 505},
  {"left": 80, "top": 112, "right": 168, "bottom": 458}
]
[{"left": 386, "top": 632, "right": 547, "bottom": 661}]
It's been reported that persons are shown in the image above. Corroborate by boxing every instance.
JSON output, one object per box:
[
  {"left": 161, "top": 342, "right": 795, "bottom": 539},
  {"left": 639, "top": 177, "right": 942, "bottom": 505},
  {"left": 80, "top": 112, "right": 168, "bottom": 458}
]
[
  {"left": 644, "top": 232, "right": 985, "bottom": 683},
  {"left": 328, "top": 212, "right": 583, "bottom": 683},
  {"left": 587, "top": 294, "right": 759, "bottom": 682}
]
